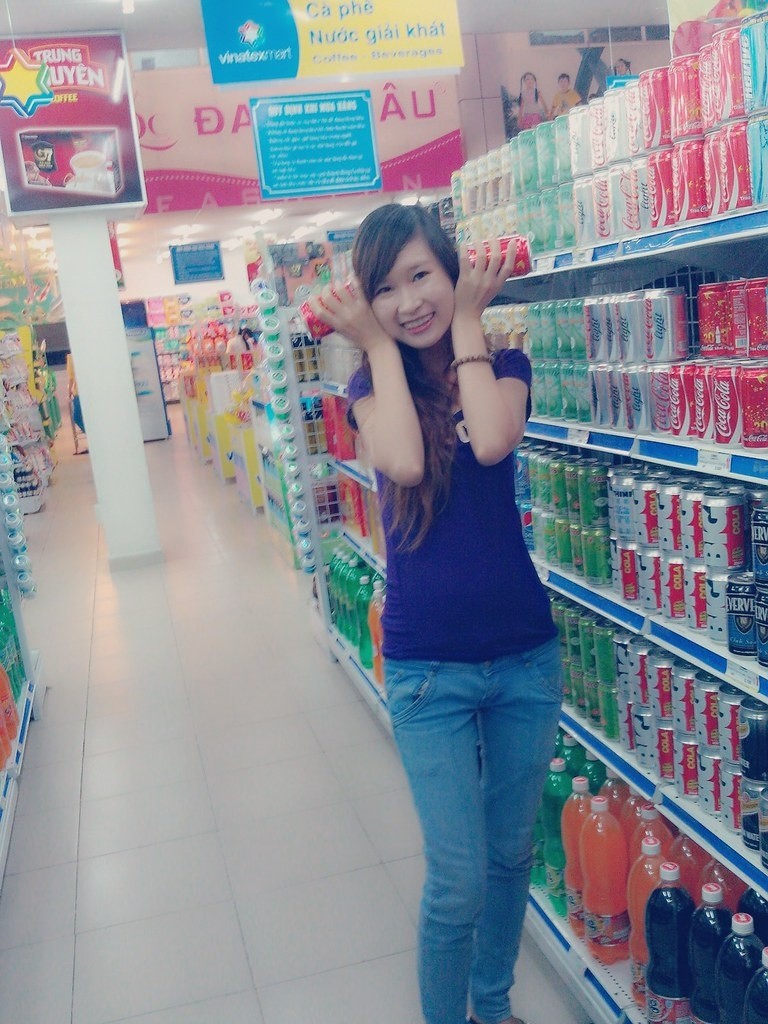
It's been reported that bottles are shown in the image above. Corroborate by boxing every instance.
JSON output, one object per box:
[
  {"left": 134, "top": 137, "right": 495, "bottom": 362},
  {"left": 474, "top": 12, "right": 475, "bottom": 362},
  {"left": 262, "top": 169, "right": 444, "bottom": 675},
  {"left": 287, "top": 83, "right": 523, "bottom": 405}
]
[
  {"left": 330, "top": 544, "right": 386, "bottom": 685},
  {"left": 530, "top": 727, "right": 767, "bottom": 1024},
  {"left": 0, "top": 588, "right": 25, "bottom": 772}
]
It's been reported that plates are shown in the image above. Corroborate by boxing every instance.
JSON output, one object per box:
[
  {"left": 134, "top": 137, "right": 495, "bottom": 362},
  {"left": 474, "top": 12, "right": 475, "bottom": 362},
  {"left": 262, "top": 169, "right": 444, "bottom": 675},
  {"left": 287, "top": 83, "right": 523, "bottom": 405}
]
[{"left": 65, "top": 171, "right": 115, "bottom": 194}]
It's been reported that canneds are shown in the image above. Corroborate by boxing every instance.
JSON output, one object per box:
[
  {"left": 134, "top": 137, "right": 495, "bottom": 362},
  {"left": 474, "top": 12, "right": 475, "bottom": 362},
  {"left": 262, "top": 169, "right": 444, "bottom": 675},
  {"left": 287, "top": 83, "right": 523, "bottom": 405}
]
[
  {"left": 294, "top": 280, "right": 357, "bottom": 342},
  {"left": 448, "top": 8, "right": 768, "bottom": 871}
]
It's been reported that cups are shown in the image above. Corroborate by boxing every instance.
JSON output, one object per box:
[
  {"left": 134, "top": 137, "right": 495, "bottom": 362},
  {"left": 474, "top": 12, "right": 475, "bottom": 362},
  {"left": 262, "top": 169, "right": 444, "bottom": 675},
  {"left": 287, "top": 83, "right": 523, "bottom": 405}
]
[{"left": 70, "top": 150, "right": 106, "bottom": 189}]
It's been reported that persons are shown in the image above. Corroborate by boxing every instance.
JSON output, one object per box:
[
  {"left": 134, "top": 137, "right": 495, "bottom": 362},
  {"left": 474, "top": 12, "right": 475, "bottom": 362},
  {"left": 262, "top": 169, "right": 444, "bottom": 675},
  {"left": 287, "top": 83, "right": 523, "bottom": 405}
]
[
  {"left": 516, "top": 72, "right": 550, "bottom": 133},
  {"left": 227, "top": 328, "right": 257, "bottom": 353},
  {"left": 66, "top": 353, "right": 89, "bottom": 455},
  {"left": 303, "top": 200, "right": 569, "bottom": 1024},
  {"left": 612, "top": 58, "right": 632, "bottom": 76},
  {"left": 550, "top": 73, "right": 584, "bottom": 121}
]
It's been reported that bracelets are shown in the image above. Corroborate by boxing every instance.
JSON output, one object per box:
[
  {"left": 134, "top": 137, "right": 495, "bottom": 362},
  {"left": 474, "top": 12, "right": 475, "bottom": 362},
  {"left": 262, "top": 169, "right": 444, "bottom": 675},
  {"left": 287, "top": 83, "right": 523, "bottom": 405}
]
[{"left": 448, "top": 354, "right": 495, "bottom": 370}]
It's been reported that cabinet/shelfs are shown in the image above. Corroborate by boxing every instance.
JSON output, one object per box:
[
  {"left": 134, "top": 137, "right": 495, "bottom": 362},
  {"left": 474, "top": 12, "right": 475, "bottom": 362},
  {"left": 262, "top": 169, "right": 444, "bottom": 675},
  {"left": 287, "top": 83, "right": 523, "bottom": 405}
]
[
  {"left": 1, "top": 348, "right": 60, "bottom": 518},
  {"left": 151, "top": 204, "right": 768, "bottom": 1024}
]
